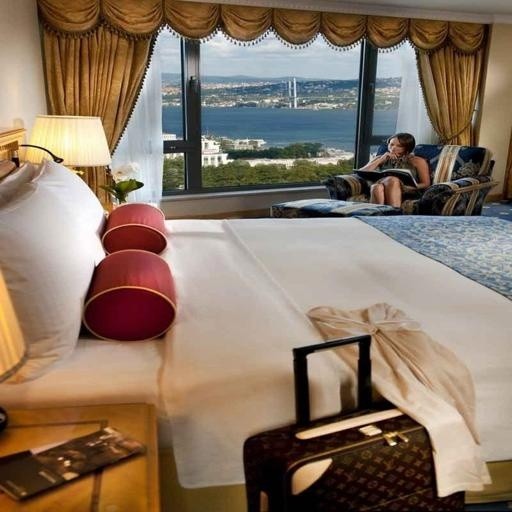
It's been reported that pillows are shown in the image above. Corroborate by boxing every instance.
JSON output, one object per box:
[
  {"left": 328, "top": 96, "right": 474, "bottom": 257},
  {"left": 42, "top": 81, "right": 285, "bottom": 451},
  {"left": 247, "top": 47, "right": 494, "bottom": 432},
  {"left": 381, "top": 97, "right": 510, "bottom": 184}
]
[{"left": 0, "top": 158, "right": 178, "bottom": 380}]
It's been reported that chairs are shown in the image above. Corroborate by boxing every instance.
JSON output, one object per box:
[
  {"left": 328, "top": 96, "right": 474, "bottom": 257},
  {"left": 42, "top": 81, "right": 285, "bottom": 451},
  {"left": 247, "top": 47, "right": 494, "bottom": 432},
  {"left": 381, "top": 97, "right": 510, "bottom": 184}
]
[{"left": 319, "top": 143, "right": 497, "bottom": 216}]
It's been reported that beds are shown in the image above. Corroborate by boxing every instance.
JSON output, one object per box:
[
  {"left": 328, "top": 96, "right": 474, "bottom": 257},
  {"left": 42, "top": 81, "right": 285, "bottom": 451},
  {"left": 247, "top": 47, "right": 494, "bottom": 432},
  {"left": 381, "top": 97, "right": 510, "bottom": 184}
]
[{"left": 0, "top": 127, "right": 512, "bottom": 511}]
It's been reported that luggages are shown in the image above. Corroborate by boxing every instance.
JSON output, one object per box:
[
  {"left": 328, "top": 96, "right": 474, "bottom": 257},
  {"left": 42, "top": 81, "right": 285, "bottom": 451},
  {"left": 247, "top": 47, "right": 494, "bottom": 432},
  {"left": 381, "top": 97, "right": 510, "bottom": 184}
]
[{"left": 243, "top": 334, "right": 465, "bottom": 512}]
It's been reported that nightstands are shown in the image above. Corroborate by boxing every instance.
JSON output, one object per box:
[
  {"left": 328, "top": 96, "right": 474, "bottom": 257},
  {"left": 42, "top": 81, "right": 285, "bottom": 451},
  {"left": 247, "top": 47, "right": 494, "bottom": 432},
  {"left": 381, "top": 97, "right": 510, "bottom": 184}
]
[{"left": 0, "top": 402, "right": 160, "bottom": 510}]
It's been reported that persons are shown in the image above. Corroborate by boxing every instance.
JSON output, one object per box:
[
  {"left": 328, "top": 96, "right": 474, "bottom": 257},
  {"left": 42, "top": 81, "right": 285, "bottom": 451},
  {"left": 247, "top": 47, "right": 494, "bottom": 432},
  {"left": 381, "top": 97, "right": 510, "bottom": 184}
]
[{"left": 358, "top": 134, "right": 432, "bottom": 210}]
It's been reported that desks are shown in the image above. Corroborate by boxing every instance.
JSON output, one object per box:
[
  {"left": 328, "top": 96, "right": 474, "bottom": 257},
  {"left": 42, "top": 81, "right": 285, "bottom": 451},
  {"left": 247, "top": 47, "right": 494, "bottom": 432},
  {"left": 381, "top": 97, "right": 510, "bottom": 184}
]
[{"left": 270, "top": 196, "right": 404, "bottom": 219}]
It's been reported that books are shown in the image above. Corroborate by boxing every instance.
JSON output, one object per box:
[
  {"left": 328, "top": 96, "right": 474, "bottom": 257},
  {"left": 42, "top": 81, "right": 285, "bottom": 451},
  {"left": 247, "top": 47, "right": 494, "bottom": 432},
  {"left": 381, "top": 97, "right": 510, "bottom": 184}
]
[
  {"left": 354, "top": 167, "right": 420, "bottom": 189},
  {"left": 1, "top": 424, "right": 146, "bottom": 502}
]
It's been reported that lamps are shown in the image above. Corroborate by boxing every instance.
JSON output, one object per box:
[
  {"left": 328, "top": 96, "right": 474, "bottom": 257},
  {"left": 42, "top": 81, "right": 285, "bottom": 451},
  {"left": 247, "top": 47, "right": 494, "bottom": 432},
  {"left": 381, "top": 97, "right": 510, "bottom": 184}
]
[
  {"left": 25, "top": 115, "right": 112, "bottom": 174},
  {"left": 0, "top": 273, "right": 33, "bottom": 392}
]
